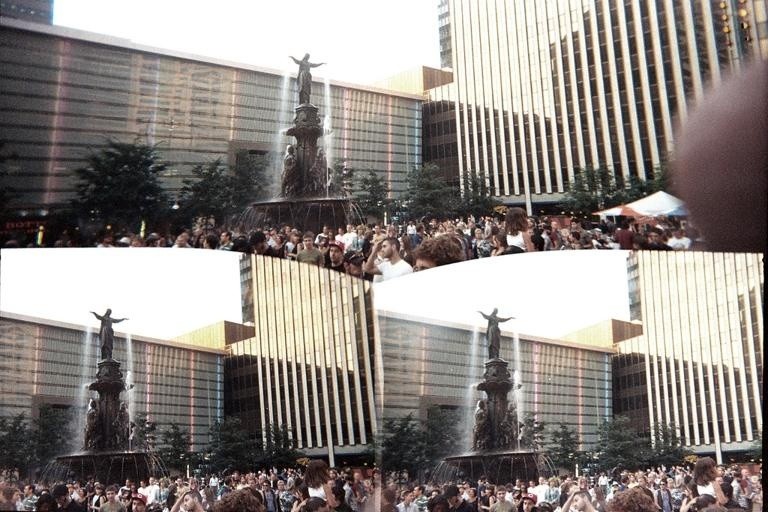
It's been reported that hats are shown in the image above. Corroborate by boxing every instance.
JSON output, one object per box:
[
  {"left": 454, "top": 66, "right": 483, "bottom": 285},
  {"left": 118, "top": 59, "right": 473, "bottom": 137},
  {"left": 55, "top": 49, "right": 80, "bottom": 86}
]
[
  {"left": 522, "top": 493, "right": 538, "bottom": 503},
  {"left": 329, "top": 241, "right": 345, "bottom": 251},
  {"left": 131, "top": 493, "right": 147, "bottom": 505}
]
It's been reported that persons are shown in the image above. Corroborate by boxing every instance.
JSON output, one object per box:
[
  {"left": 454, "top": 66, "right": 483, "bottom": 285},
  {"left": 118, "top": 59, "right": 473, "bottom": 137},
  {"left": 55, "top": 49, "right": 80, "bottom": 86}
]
[
  {"left": 279, "top": 145, "right": 297, "bottom": 197},
  {"left": 477, "top": 308, "right": 517, "bottom": 358},
  {"left": 470, "top": 400, "right": 491, "bottom": 451},
  {"left": 0, "top": 455, "right": 762, "bottom": 511},
  {"left": 80, "top": 399, "right": 98, "bottom": 449},
  {"left": 90, "top": 308, "right": 129, "bottom": 360},
  {"left": 286, "top": 51, "right": 326, "bottom": 103},
  {"left": 0, "top": 206, "right": 767, "bottom": 282}
]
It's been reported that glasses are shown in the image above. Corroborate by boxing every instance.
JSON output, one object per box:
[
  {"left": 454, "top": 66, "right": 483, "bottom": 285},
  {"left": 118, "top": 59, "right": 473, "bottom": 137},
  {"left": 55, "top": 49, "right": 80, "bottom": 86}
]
[{"left": 659, "top": 483, "right": 666, "bottom": 486}]
